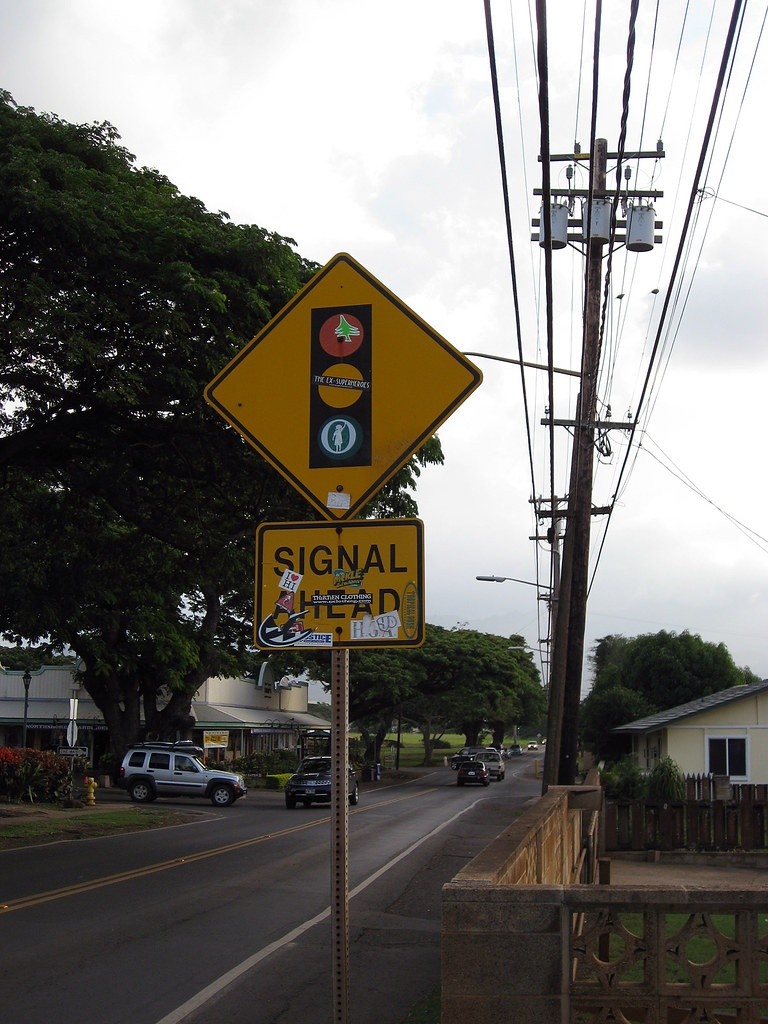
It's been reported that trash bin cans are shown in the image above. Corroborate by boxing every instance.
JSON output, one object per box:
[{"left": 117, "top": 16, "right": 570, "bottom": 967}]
[{"left": 371, "top": 763, "right": 381, "bottom": 781}]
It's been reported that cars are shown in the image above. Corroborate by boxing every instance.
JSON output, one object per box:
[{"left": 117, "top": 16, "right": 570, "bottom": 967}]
[
  {"left": 527, "top": 741, "right": 539, "bottom": 750},
  {"left": 510, "top": 745, "right": 523, "bottom": 755},
  {"left": 488, "top": 743, "right": 512, "bottom": 760},
  {"left": 456, "top": 760, "right": 491, "bottom": 787},
  {"left": 542, "top": 739, "right": 547, "bottom": 745}
]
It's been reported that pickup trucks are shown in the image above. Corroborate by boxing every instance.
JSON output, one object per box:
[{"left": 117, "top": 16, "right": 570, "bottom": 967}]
[{"left": 473, "top": 752, "right": 505, "bottom": 780}]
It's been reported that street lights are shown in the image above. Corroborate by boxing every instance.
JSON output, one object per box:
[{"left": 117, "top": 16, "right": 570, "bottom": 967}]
[
  {"left": 22, "top": 668, "right": 33, "bottom": 748},
  {"left": 475, "top": 575, "right": 561, "bottom": 669}
]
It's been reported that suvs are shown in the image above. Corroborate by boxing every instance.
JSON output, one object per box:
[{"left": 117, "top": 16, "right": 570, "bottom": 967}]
[
  {"left": 284, "top": 755, "right": 360, "bottom": 808},
  {"left": 451, "top": 747, "right": 498, "bottom": 770},
  {"left": 118, "top": 740, "right": 248, "bottom": 807}
]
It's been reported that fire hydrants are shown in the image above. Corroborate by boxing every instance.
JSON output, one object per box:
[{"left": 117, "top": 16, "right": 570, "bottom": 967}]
[{"left": 86, "top": 777, "right": 98, "bottom": 806}]
